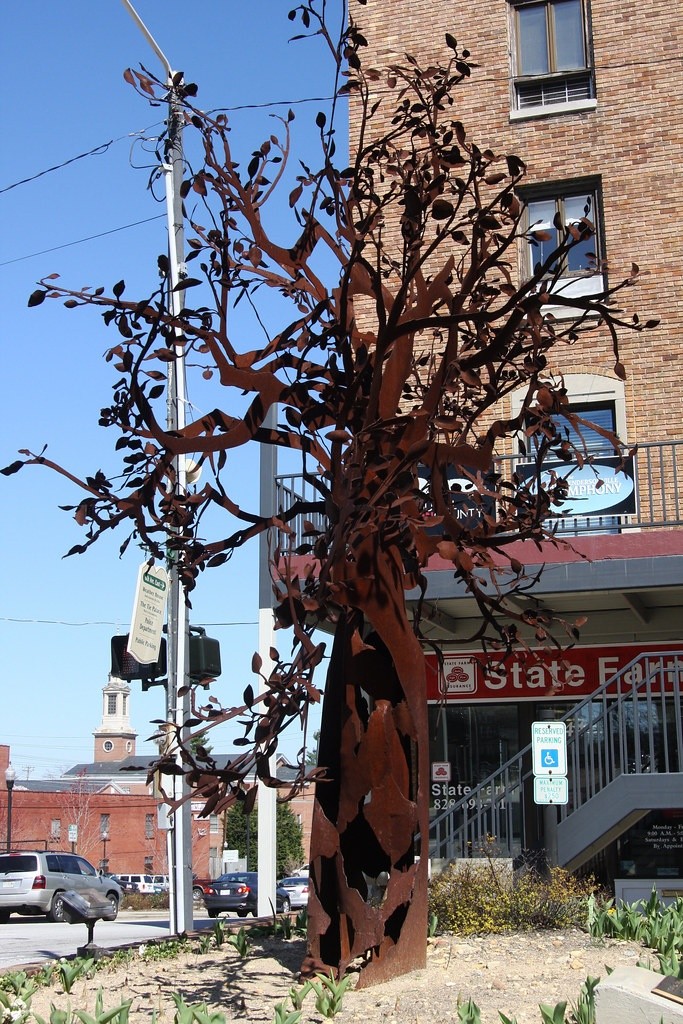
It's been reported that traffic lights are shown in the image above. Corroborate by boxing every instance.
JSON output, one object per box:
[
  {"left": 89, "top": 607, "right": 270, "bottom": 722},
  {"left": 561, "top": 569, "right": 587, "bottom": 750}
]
[{"left": 110, "top": 633, "right": 168, "bottom": 680}]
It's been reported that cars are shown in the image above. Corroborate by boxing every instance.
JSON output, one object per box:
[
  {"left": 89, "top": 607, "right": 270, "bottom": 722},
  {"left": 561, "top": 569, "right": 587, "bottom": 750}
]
[
  {"left": 204, "top": 871, "right": 292, "bottom": 918},
  {"left": 290, "top": 863, "right": 311, "bottom": 878},
  {"left": 278, "top": 877, "right": 310, "bottom": 909}
]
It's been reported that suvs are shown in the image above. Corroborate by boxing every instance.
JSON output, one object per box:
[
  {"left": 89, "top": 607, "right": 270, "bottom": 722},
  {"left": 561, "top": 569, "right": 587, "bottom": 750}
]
[
  {"left": 0, "top": 849, "right": 124, "bottom": 924},
  {"left": 107, "top": 872, "right": 216, "bottom": 901}
]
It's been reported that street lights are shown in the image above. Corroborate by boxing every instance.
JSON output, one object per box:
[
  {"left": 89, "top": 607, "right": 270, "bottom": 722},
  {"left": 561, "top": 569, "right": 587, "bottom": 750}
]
[
  {"left": 98, "top": 830, "right": 111, "bottom": 876},
  {"left": 4, "top": 761, "right": 16, "bottom": 852}
]
